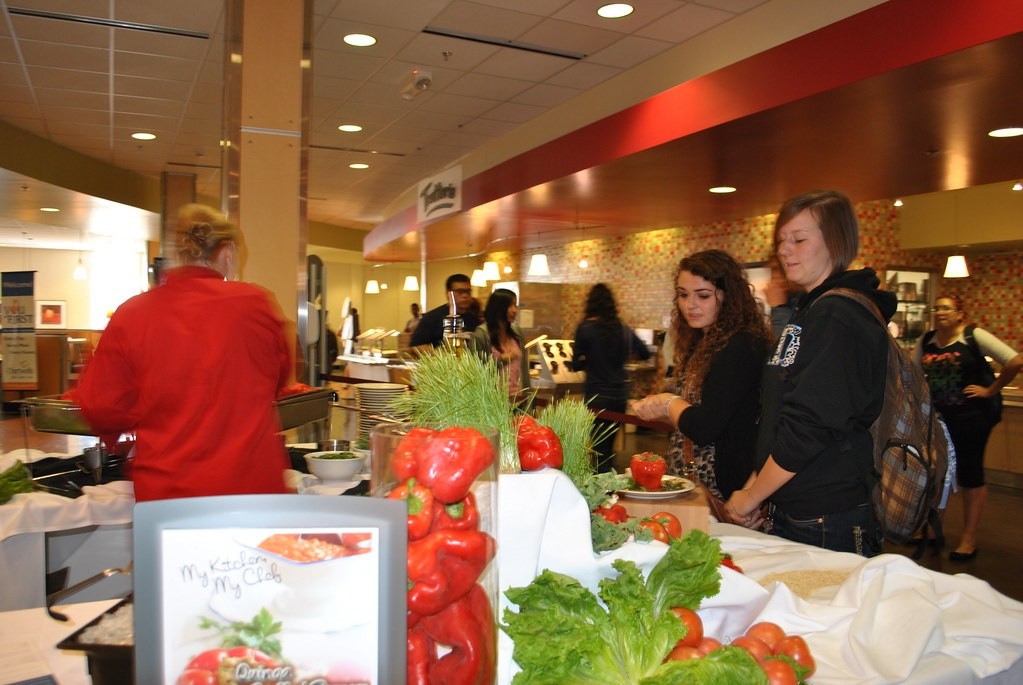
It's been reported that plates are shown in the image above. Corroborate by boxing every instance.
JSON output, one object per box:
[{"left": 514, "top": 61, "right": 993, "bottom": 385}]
[
  {"left": 612, "top": 475, "right": 696, "bottom": 500},
  {"left": 354, "top": 383, "right": 410, "bottom": 450},
  {"left": 237, "top": 534, "right": 373, "bottom": 566}
]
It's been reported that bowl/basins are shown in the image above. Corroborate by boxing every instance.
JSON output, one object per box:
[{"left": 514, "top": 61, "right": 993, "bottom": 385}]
[
  {"left": 352, "top": 447, "right": 372, "bottom": 472},
  {"left": 303, "top": 450, "right": 366, "bottom": 485}
]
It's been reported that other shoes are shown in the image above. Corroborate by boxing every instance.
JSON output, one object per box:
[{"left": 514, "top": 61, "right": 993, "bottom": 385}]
[{"left": 950, "top": 546, "right": 977, "bottom": 562}]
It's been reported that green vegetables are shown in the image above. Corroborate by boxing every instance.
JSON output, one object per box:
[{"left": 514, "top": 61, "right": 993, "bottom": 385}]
[
  {"left": 198, "top": 607, "right": 285, "bottom": 656},
  {"left": 312, "top": 451, "right": 356, "bottom": 459},
  {"left": 580, "top": 467, "right": 670, "bottom": 554},
  {"left": 0, "top": 459, "right": 37, "bottom": 504}
]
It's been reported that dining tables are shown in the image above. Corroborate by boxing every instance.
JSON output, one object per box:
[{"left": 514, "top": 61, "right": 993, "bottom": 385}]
[{"left": 0, "top": 441, "right": 1023, "bottom": 685}]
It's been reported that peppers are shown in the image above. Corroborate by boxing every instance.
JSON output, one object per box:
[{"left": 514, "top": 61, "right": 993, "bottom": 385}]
[
  {"left": 177, "top": 647, "right": 294, "bottom": 685},
  {"left": 384, "top": 426, "right": 499, "bottom": 685},
  {"left": 630, "top": 451, "right": 667, "bottom": 494},
  {"left": 512, "top": 414, "right": 564, "bottom": 470}
]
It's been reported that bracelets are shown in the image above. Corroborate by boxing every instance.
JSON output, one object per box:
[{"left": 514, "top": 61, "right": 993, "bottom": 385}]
[{"left": 663, "top": 395, "right": 684, "bottom": 418}]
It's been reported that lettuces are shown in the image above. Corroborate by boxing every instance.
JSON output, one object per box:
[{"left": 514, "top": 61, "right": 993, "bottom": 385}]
[
  {"left": 500, "top": 523, "right": 766, "bottom": 685},
  {"left": 765, "top": 651, "right": 810, "bottom": 685}
]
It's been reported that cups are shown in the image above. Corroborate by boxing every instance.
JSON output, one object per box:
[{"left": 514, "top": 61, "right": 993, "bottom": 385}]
[
  {"left": 318, "top": 439, "right": 350, "bottom": 452},
  {"left": 370, "top": 422, "right": 416, "bottom": 499}
]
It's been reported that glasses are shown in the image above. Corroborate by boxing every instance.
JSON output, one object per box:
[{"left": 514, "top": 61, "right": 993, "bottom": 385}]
[
  {"left": 452, "top": 287, "right": 472, "bottom": 294},
  {"left": 930, "top": 306, "right": 957, "bottom": 314}
]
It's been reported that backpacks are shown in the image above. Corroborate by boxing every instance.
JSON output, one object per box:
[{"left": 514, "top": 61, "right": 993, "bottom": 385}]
[{"left": 811, "top": 289, "right": 949, "bottom": 538}]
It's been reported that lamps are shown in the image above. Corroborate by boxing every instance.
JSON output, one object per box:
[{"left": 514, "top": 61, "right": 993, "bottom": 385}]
[
  {"left": 528, "top": 254, "right": 550, "bottom": 275},
  {"left": 365, "top": 280, "right": 379, "bottom": 293},
  {"left": 403, "top": 276, "right": 419, "bottom": 291},
  {"left": 943, "top": 256, "right": 969, "bottom": 277},
  {"left": 482, "top": 261, "right": 500, "bottom": 280},
  {"left": 470, "top": 269, "right": 486, "bottom": 287}
]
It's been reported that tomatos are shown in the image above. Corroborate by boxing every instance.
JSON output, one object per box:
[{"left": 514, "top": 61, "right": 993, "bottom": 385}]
[
  {"left": 594, "top": 503, "right": 683, "bottom": 545},
  {"left": 658, "top": 606, "right": 815, "bottom": 685}
]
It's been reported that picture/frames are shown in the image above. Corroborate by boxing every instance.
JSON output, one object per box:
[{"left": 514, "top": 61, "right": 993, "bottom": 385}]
[{"left": 33, "top": 300, "right": 67, "bottom": 329}]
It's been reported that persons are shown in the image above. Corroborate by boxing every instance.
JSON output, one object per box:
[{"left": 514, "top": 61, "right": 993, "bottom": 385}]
[
  {"left": 410, "top": 274, "right": 483, "bottom": 347},
  {"left": 910, "top": 292, "right": 1023, "bottom": 564},
  {"left": 724, "top": 188, "right": 898, "bottom": 557},
  {"left": 75, "top": 203, "right": 299, "bottom": 502},
  {"left": 572, "top": 284, "right": 651, "bottom": 473},
  {"left": 469, "top": 289, "right": 531, "bottom": 416},
  {"left": 404, "top": 302, "right": 426, "bottom": 332},
  {"left": 629, "top": 249, "right": 781, "bottom": 533}
]
